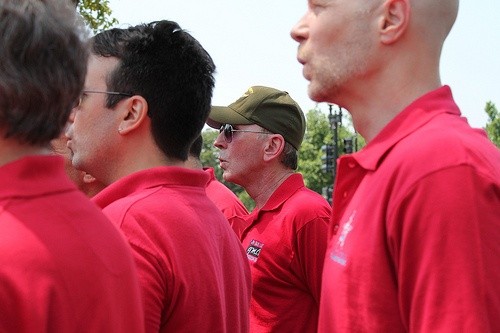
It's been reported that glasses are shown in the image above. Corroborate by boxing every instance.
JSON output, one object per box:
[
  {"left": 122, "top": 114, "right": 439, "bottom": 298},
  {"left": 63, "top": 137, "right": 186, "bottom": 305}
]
[
  {"left": 220, "top": 124, "right": 273, "bottom": 143},
  {"left": 73, "top": 88, "right": 152, "bottom": 118}
]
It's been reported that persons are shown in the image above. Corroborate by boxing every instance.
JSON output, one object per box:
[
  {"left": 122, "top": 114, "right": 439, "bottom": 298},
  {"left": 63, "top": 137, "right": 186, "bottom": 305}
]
[
  {"left": 0, "top": 0, "right": 333, "bottom": 333},
  {"left": 289, "top": 0, "right": 500, "bottom": 333}
]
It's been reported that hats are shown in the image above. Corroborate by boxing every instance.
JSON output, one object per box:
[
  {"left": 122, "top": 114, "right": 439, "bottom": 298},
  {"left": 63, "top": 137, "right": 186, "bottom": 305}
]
[{"left": 206, "top": 86, "right": 306, "bottom": 150}]
